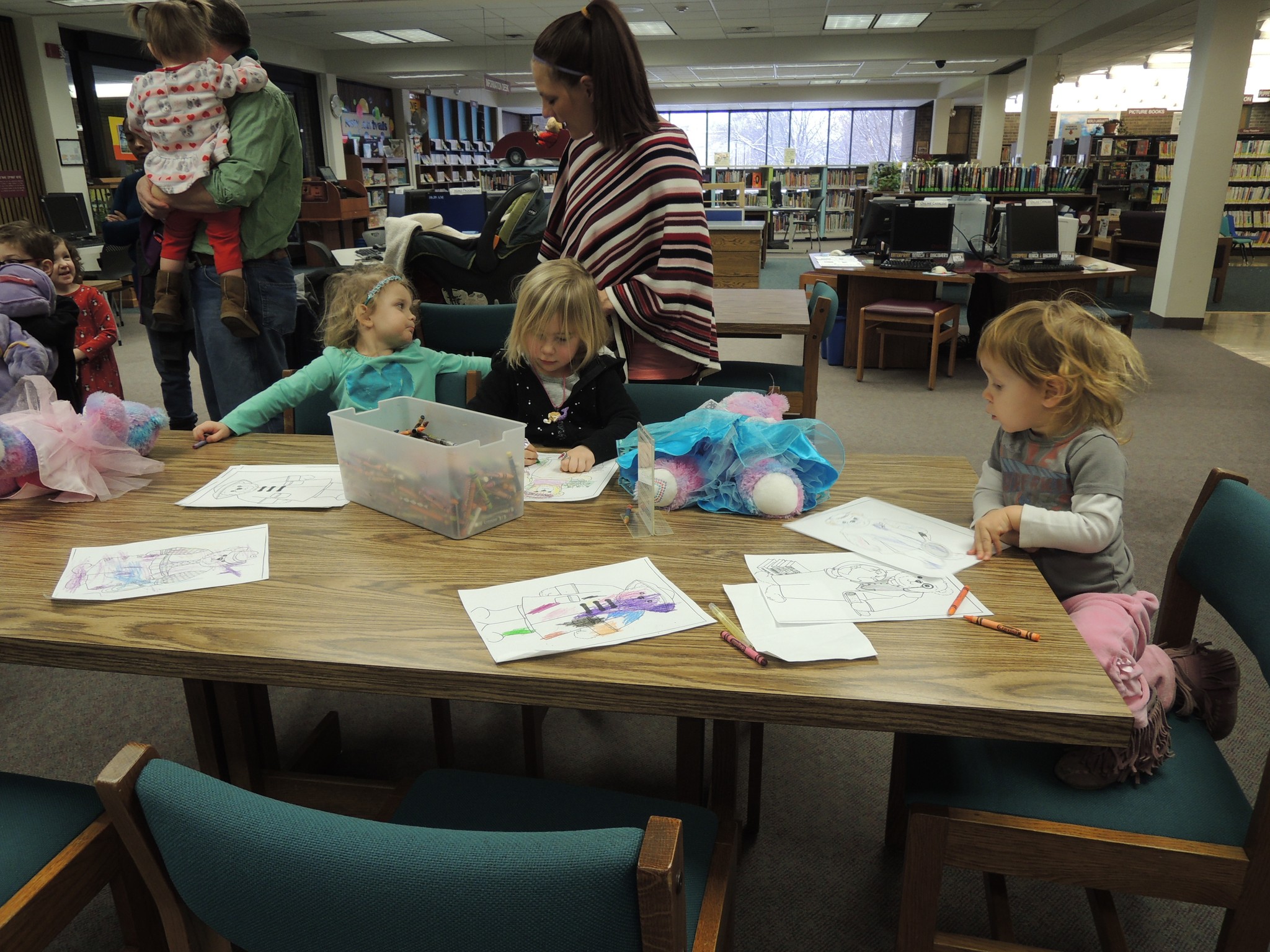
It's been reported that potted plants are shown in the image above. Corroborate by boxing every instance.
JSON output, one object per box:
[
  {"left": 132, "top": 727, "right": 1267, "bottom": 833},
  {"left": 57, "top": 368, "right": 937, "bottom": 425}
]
[{"left": 1101, "top": 119, "right": 1141, "bottom": 138}]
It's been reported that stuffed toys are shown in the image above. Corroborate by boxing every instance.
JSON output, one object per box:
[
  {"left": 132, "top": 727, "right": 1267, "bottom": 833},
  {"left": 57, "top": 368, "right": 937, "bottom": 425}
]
[
  {"left": 615, "top": 373, "right": 847, "bottom": 522},
  {"left": 1, "top": 389, "right": 172, "bottom": 496}
]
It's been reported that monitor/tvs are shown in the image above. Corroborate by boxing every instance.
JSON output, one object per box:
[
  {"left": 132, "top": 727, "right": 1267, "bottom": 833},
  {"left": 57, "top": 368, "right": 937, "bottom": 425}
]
[
  {"left": 38, "top": 195, "right": 90, "bottom": 240},
  {"left": 47, "top": 191, "right": 93, "bottom": 233},
  {"left": 1006, "top": 202, "right": 1060, "bottom": 261},
  {"left": 723, "top": 189, "right": 737, "bottom": 206},
  {"left": 856, "top": 199, "right": 911, "bottom": 265},
  {"left": 889, "top": 206, "right": 953, "bottom": 259},
  {"left": 319, "top": 165, "right": 339, "bottom": 186},
  {"left": 770, "top": 181, "right": 783, "bottom": 208}
]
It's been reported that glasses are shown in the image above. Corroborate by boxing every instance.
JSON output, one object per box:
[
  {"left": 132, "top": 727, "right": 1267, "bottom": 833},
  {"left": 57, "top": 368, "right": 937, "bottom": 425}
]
[{"left": 0, "top": 258, "right": 45, "bottom": 266}]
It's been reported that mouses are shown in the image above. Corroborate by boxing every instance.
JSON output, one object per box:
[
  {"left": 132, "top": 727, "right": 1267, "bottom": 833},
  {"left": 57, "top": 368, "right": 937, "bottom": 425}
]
[
  {"left": 1087, "top": 262, "right": 1108, "bottom": 270},
  {"left": 829, "top": 250, "right": 846, "bottom": 256},
  {"left": 930, "top": 266, "right": 947, "bottom": 274}
]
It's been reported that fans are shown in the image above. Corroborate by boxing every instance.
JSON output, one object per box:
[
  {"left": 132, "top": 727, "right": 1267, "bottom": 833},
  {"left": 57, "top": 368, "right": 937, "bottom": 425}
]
[{"left": 407, "top": 107, "right": 429, "bottom": 136}]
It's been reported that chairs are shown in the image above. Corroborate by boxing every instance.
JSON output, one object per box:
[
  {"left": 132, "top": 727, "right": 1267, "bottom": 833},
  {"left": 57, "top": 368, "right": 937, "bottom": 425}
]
[
  {"left": 0, "top": 771, "right": 170, "bottom": 951},
  {"left": 799, "top": 270, "right": 839, "bottom": 302},
  {"left": 94, "top": 743, "right": 745, "bottom": 952},
  {"left": 81, "top": 241, "right": 137, "bottom": 327},
  {"left": 1225, "top": 213, "right": 1260, "bottom": 261},
  {"left": 702, "top": 181, "right": 745, "bottom": 209},
  {"left": 1220, "top": 216, "right": 1252, "bottom": 264},
  {"left": 705, "top": 208, "right": 745, "bottom": 222},
  {"left": 362, "top": 191, "right": 413, "bottom": 247},
  {"left": 781, "top": 196, "right": 825, "bottom": 252},
  {"left": 426, "top": 190, "right": 487, "bottom": 235},
  {"left": 880, "top": 467, "right": 1270, "bottom": 952},
  {"left": 625, "top": 384, "right": 782, "bottom": 426},
  {"left": 79, "top": 281, "right": 123, "bottom": 348},
  {"left": 411, "top": 301, "right": 518, "bottom": 358},
  {"left": 282, "top": 369, "right": 482, "bottom": 438},
  {"left": 306, "top": 240, "right": 336, "bottom": 268},
  {"left": 694, "top": 279, "right": 840, "bottom": 445}
]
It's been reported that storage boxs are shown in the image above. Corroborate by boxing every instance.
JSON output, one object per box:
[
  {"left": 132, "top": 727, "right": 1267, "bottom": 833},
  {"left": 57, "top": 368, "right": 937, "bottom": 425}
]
[
  {"left": 819, "top": 299, "right": 847, "bottom": 367},
  {"left": 328, "top": 395, "right": 528, "bottom": 540},
  {"left": 923, "top": 197, "right": 991, "bottom": 253}
]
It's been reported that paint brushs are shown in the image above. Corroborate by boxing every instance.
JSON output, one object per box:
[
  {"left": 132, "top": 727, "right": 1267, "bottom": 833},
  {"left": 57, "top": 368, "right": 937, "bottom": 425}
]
[
  {"left": 192, "top": 440, "right": 207, "bottom": 450},
  {"left": 947, "top": 585, "right": 970, "bottom": 616},
  {"left": 623, "top": 505, "right": 632, "bottom": 525},
  {"left": 338, "top": 414, "right": 523, "bottom": 538},
  {"left": 720, "top": 630, "right": 768, "bottom": 667},
  {"left": 963, "top": 614, "right": 1040, "bottom": 643}
]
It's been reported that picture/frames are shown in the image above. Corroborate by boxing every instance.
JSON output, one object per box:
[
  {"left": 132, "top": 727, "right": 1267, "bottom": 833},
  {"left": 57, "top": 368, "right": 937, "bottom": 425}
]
[{"left": 56, "top": 139, "right": 86, "bottom": 166}]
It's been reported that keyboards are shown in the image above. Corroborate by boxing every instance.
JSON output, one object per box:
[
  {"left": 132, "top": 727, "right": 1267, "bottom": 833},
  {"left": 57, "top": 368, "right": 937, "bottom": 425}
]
[
  {"left": 1008, "top": 264, "right": 1084, "bottom": 272},
  {"left": 879, "top": 258, "right": 958, "bottom": 273},
  {"left": 843, "top": 247, "right": 874, "bottom": 255},
  {"left": 64, "top": 237, "right": 107, "bottom": 248}
]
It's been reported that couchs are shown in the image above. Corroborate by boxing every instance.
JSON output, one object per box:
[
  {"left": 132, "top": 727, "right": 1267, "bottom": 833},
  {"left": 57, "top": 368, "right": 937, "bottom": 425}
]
[{"left": 1104, "top": 210, "right": 1233, "bottom": 303}]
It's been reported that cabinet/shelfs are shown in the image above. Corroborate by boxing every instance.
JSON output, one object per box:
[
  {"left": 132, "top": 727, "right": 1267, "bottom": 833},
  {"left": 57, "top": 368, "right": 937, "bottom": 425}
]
[
  {"left": 475, "top": 165, "right": 559, "bottom": 215},
  {"left": 1050, "top": 138, "right": 1079, "bottom": 169},
  {"left": 409, "top": 135, "right": 496, "bottom": 190},
  {"left": 344, "top": 154, "right": 411, "bottom": 231},
  {"left": 1075, "top": 134, "right": 1270, "bottom": 250},
  {"left": 699, "top": 165, "right": 868, "bottom": 240}
]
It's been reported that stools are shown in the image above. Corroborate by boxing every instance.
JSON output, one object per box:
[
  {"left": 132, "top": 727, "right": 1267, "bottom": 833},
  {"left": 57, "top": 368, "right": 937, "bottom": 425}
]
[
  {"left": 1079, "top": 305, "right": 1135, "bottom": 341},
  {"left": 857, "top": 298, "right": 961, "bottom": 391}
]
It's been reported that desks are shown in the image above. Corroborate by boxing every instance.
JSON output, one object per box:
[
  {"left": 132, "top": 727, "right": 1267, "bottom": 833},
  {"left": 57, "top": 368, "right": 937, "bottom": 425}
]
[
  {"left": 75, "top": 243, "right": 131, "bottom": 327},
  {"left": 706, "top": 220, "right": 766, "bottom": 289},
  {"left": 810, "top": 244, "right": 1136, "bottom": 369},
  {"left": 852, "top": 185, "right": 1100, "bottom": 258},
  {"left": 296, "top": 213, "right": 377, "bottom": 265},
  {"left": 709, "top": 288, "right": 812, "bottom": 340},
  {"left": 331, "top": 247, "right": 386, "bottom": 271},
  {"left": 0, "top": 429, "right": 1135, "bottom": 864},
  {"left": 703, "top": 206, "right": 771, "bottom": 268}
]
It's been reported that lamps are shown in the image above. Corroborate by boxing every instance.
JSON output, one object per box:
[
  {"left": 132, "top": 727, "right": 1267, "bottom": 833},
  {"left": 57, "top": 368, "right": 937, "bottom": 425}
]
[
  {"left": 454, "top": 83, "right": 461, "bottom": 95},
  {"left": 1005, "top": 9, "right": 1270, "bottom": 114},
  {"left": 425, "top": 85, "right": 431, "bottom": 96}
]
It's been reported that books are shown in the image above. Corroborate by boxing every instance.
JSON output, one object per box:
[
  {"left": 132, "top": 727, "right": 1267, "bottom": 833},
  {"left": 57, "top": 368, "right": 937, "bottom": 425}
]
[
  {"left": 482, "top": 172, "right": 558, "bottom": 190},
  {"left": 869, "top": 158, "right": 1092, "bottom": 193},
  {"left": 353, "top": 138, "right": 408, "bottom": 227},
  {"left": 1045, "top": 138, "right": 1269, "bottom": 248},
  {"left": 700, "top": 167, "right": 868, "bottom": 229}
]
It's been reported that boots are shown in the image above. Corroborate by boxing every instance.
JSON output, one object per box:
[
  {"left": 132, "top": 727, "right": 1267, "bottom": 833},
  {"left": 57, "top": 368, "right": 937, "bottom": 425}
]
[
  {"left": 151, "top": 270, "right": 184, "bottom": 326},
  {"left": 219, "top": 274, "right": 260, "bottom": 338},
  {"left": 1163, "top": 648, "right": 1241, "bottom": 742},
  {"left": 1053, "top": 682, "right": 1168, "bottom": 790}
]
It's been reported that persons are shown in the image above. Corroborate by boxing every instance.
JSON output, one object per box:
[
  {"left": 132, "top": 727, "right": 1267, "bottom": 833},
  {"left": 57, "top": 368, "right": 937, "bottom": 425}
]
[
  {"left": 46, "top": 233, "right": 126, "bottom": 408},
  {"left": 967, "top": 289, "right": 1243, "bottom": 789},
  {"left": 187, "top": 259, "right": 493, "bottom": 442},
  {"left": 137, "top": 1, "right": 303, "bottom": 431},
  {"left": 0, "top": 216, "right": 82, "bottom": 414},
  {"left": 469, "top": 257, "right": 639, "bottom": 474},
  {"left": 124, "top": 1, "right": 262, "bottom": 340},
  {"left": 101, "top": 115, "right": 201, "bottom": 429},
  {"left": 531, "top": 0, "right": 722, "bottom": 384}
]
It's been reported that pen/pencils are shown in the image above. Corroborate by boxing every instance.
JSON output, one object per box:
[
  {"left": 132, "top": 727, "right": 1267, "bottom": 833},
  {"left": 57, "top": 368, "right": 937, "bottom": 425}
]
[
  {"left": 354, "top": 258, "right": 364, "bottom": 261},
  {"left": 356, "top": 253, "right": 364, "bottom": 259},
  {"left": 708, "top": 602, "right": 753, "bottom": 649}
]
